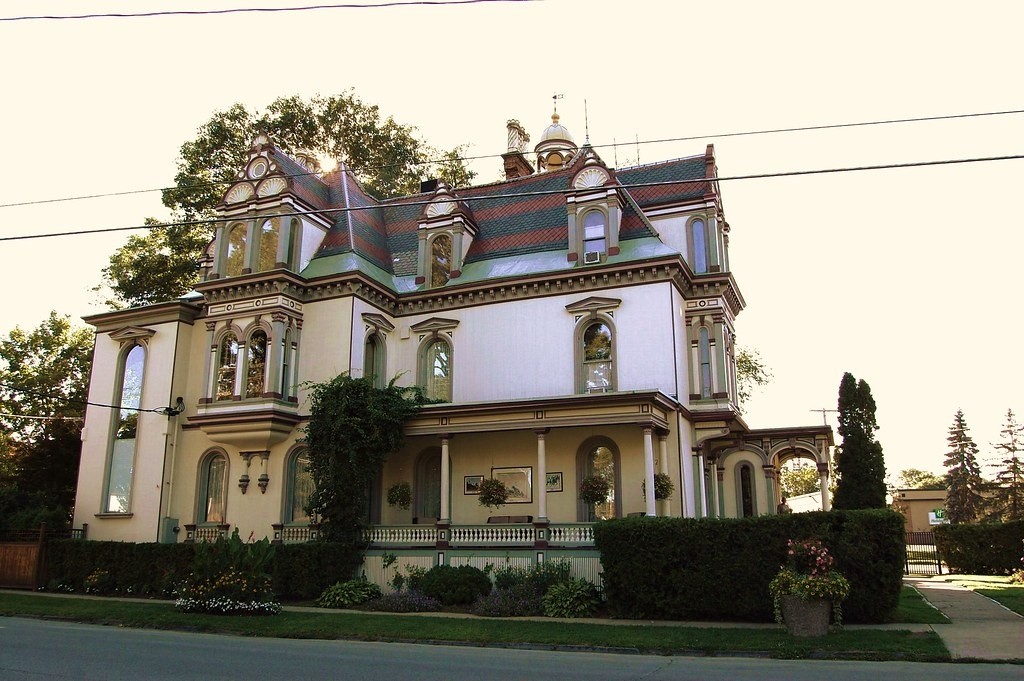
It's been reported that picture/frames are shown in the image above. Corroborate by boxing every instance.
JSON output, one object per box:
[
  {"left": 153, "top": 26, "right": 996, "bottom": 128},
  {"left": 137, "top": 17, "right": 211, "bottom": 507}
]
[
  {"left": 546, "top": 472, "right": 563, "bottom": 492},
  {"left": 464, "top": 475, "right": 484, "bottom": 495},
  {"left": 491, "top": 466, "right": 533, "bottom": 504}
]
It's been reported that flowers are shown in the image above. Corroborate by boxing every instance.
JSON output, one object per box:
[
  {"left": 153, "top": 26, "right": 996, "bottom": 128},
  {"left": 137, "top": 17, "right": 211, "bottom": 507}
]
[{"left": 769, "top": 535, "right": 849, "bottom": 635}]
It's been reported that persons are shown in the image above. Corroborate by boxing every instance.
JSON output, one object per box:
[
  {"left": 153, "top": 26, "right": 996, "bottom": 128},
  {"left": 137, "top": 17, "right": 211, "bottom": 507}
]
[{"left": 778, "top": 497, "right": 793, "bottom": 514}]
